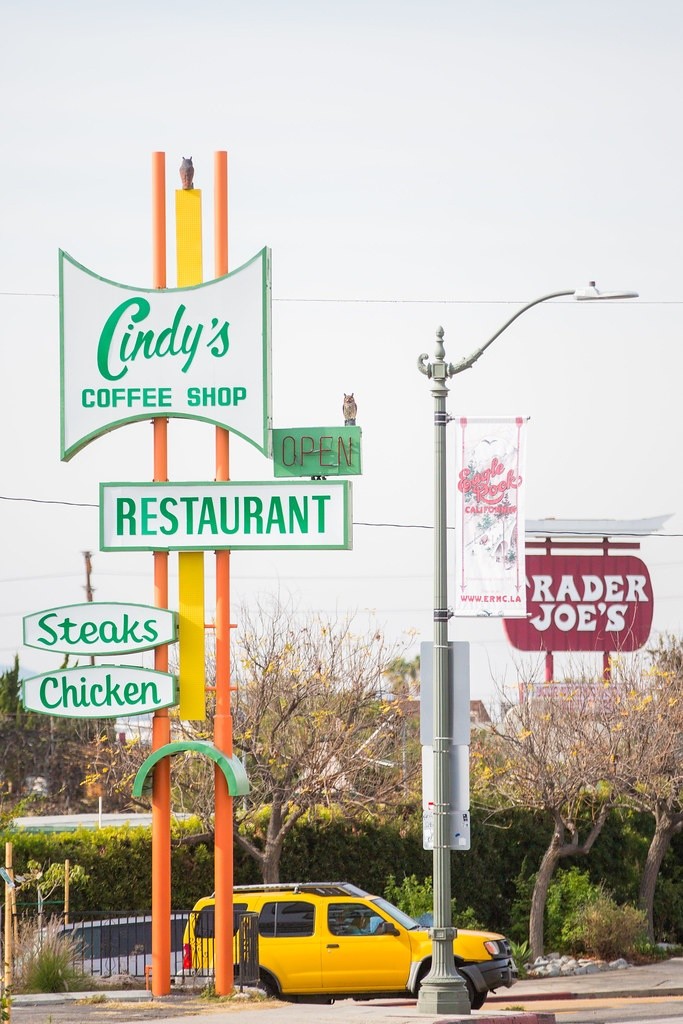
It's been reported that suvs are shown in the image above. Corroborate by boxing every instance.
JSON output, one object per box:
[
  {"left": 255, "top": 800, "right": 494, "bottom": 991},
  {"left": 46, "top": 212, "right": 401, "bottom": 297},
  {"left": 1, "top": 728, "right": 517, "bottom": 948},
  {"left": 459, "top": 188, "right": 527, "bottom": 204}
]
[
  {"left": 14, "top": 912, "right": 201, "bottom": 993},
  {"left": 180, "top": 881, "right": 520, "bottom": 1012}
]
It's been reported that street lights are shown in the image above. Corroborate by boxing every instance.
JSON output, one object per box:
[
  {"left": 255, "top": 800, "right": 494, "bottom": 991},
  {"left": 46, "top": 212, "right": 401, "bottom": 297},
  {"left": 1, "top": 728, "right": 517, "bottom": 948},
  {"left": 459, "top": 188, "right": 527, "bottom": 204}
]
[{"left": 410, "top": 280, "right": 642, "bottom": 1014}]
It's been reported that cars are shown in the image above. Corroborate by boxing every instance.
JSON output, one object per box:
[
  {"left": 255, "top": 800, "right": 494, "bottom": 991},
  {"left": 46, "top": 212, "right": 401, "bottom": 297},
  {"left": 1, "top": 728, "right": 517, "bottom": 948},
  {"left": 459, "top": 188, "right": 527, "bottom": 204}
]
[{"left": 111, "top": 693, "right": 352, "bottom": 798}]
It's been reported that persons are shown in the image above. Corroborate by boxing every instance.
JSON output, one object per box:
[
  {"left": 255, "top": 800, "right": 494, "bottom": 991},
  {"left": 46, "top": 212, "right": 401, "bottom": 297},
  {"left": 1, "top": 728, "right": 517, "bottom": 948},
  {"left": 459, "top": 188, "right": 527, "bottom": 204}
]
[{"left": 345, "top": 915, "right": 385, "bottom": 934}]
